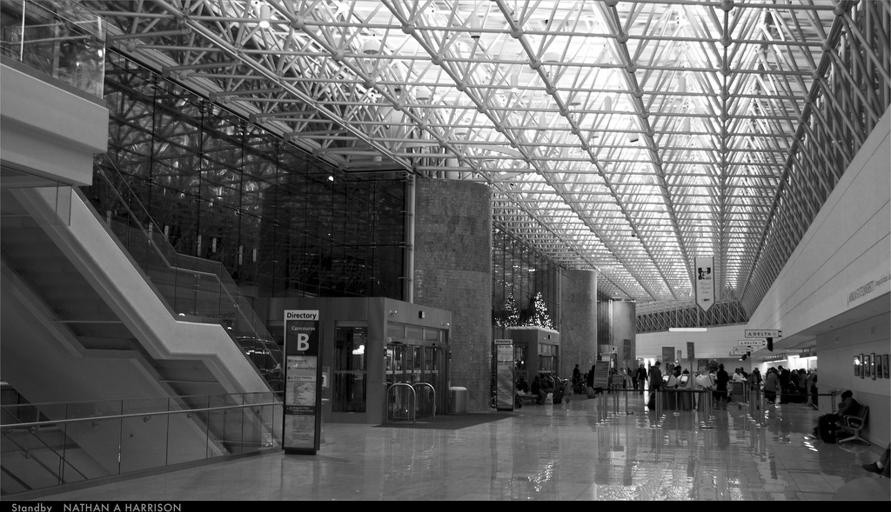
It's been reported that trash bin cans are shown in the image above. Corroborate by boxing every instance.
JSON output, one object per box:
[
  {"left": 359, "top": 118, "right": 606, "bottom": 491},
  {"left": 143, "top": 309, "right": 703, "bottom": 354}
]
[{"left": 447, "top": 386, "right": 468, "bottom": 416}]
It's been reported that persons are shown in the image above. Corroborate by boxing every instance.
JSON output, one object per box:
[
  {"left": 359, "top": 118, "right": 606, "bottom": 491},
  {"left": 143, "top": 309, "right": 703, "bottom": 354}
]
[
  {"left": 863, "top": 441, "right": 891, "bottom": 477},
  {"left": 734, "top": 363, "right": 818, "bottom": 402},
  {"left": 516, "top": 359, "right": 729, "bottom": 411},
  {"left": 820, "top": 390, "right": 859, "bottom": 443}
]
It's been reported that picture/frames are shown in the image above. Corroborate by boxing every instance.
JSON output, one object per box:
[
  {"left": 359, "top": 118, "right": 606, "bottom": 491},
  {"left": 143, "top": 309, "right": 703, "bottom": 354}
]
[{"left": 852, "top": 352, "right": 890, "bottom": 380}]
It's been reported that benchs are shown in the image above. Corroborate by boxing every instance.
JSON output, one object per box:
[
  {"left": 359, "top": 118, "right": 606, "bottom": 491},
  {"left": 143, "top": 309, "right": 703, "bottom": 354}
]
[
  {"left": 830, "top": 401, "right": 872, "bottom": 446},
  {"left": 516, "top": 374, "right": 599, "bottom": 405}
]
